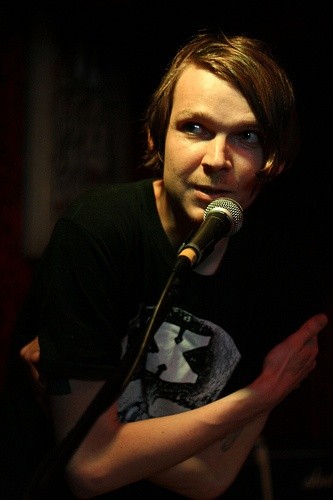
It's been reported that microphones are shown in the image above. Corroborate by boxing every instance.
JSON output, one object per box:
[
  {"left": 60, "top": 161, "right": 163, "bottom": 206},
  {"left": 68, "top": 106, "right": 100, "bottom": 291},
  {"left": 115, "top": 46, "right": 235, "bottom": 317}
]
[{"left": 169, "top": 198, "right": 246, "bottom": 276}]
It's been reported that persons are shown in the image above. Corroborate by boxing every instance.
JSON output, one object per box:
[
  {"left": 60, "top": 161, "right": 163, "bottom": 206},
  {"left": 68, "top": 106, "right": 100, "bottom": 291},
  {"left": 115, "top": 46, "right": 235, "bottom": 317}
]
[{"left": 20, "top": 33, "right": 328, "bottom": 500}]
